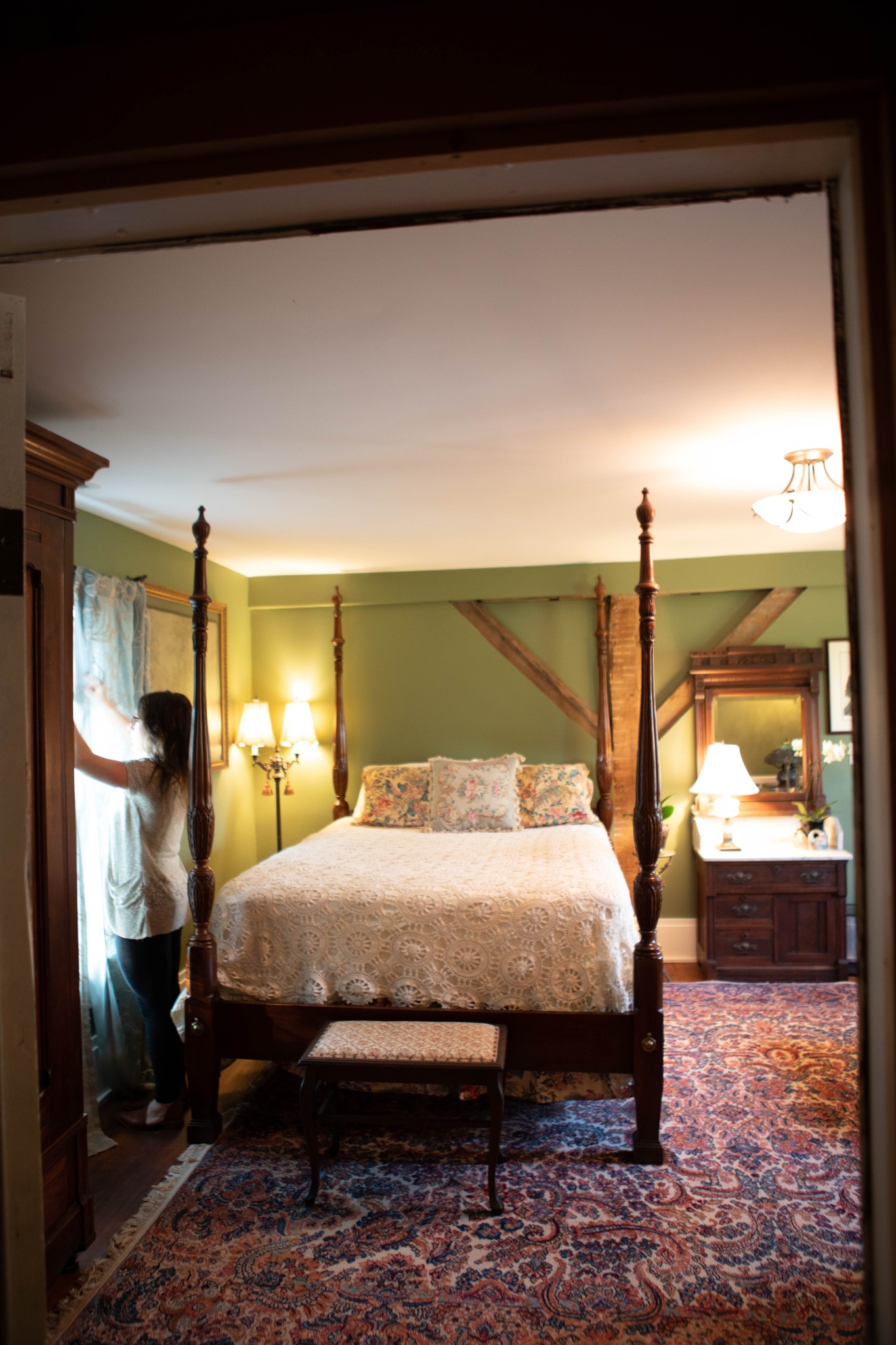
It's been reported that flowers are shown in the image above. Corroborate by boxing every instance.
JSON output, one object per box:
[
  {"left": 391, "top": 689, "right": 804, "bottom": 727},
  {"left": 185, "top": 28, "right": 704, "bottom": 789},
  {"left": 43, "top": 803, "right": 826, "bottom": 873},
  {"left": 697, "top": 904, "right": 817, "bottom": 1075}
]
[
  {"left": 789, "top": 739, "right": 846, "bottom": 822},
  {"left": 791, "top": 739, "right": 803, "bottom": 758}
]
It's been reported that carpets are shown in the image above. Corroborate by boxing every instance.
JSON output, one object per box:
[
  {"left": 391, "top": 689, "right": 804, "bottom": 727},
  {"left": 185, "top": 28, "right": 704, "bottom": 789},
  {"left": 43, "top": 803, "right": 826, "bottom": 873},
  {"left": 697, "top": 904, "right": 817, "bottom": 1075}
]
[{"left": 43, "top": 981, "right": 867, "bottom": 1345}]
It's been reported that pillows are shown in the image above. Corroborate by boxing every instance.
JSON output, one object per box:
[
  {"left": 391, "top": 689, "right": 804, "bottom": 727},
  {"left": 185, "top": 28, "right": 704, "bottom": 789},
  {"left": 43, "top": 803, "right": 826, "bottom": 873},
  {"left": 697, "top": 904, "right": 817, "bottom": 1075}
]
[
  {"left": 348, "top": 758, "right": 431, "bottom": 826},
  {"left": 705, "top": 684, "right": 812, "bottom": 803},
  {"left": 424, "top": 751, "right": 524, "bottom": 833},
  {"left": 517, "top": 762, "right": 601, "bottom": 829}
]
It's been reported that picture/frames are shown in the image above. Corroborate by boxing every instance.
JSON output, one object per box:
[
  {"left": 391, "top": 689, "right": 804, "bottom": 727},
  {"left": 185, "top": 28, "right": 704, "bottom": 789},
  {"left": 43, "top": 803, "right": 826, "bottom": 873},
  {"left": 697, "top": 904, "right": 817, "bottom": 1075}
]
[
  {"left": 826, "top": 637, "right": 855, "bottom": 737},
  {"left": 142, "top": 579, "right": 229, "bottom": 774}
]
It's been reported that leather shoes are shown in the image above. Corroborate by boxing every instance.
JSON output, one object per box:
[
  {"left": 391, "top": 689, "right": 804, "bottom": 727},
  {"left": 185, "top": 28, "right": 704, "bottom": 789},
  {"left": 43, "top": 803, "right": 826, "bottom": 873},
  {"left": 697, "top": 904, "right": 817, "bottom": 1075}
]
[{"left": 117, "top": 1088, "right": 191, "bottom": 1130}]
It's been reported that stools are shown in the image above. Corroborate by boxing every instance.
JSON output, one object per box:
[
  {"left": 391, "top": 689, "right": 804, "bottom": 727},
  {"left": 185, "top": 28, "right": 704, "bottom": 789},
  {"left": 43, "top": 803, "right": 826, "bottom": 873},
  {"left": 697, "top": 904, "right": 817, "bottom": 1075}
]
[{"left": 294, "top": 1016, "right": 506, "bottom": 1215}]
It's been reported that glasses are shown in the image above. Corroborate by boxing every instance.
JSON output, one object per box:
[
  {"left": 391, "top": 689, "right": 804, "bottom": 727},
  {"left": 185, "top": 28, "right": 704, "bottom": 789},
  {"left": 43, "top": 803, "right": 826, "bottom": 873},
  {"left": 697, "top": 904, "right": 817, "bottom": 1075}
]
[{"left": 131, "top": 717, "right": 148, "bottom": 730}]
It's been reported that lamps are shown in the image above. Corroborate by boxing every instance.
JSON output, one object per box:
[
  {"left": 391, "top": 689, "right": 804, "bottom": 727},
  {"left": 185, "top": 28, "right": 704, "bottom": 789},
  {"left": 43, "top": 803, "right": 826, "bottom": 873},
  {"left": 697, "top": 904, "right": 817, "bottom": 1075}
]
[
  {"left": 233, "top": 693, "right": 320, "bottom": 854},
  {"left": 750, "top": 448, "right": 847, "bottom": 537},
  {"left": 689, "top": 739, "right": 760, "bottom": 854}
]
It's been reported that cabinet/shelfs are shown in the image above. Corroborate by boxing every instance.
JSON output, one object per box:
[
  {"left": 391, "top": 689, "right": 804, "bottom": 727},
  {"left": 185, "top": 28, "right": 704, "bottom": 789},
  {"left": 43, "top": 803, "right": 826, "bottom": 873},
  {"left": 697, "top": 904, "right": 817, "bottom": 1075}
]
[{"left": 688, "top": 642, "right": 849, "bottom": 982}]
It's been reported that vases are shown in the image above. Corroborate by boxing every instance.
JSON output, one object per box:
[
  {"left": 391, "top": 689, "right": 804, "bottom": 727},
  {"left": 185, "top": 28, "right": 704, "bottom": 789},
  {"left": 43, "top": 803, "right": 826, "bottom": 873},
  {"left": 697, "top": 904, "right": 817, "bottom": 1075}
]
[{"left": 799, "top": 818, "right": 824, "bottom": 833}]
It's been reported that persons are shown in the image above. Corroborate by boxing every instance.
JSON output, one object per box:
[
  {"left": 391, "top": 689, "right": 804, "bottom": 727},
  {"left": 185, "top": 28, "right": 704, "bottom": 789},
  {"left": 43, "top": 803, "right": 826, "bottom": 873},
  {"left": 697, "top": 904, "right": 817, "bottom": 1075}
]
[{"left": 74, "top": 689, "right": 193, "bottom": 1137}]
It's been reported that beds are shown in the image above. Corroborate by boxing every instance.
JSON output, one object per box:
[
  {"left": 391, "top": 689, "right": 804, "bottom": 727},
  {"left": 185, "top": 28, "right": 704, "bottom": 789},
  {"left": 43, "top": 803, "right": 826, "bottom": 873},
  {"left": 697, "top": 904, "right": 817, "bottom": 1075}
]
[{"left": 181, "top": 485, "right": 667, "bottom": 1168}]
